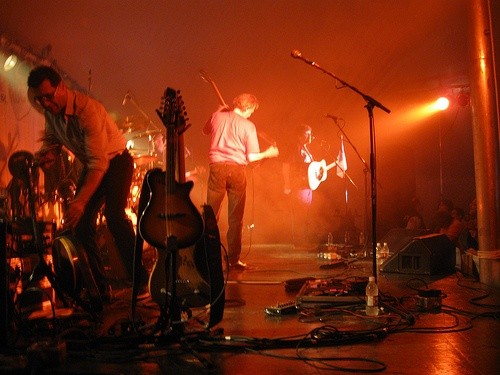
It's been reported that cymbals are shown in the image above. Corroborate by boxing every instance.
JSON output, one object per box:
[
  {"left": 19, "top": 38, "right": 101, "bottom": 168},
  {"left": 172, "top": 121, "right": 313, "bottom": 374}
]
[{"left": 122, "top": 129, "right": 162, "bottom": 139}]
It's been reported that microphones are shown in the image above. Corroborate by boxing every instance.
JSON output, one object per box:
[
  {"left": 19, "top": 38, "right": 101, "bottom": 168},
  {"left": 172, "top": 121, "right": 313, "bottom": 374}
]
[
  {"left": 122, "top": 95, "right": 128, "bottom": 105},
  {"left": 322, "top": 112, "right": 340, "bottom": 121},
  {"left": 291, "top": 49, "right": 320, "bottom": 68}
]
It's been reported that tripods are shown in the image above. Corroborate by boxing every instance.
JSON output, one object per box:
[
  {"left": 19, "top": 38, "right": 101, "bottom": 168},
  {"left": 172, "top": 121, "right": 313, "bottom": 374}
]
[{"left": 310, "top": 63, "right": 416, "bottom": 322}]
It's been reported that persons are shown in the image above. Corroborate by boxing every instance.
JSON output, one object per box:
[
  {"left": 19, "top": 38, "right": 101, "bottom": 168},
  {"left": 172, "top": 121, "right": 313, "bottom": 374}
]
[
  {"left": 400, "top": 195, "right": 479, "bottom": 282},
  {"left": 203, "top": 93, "right": 280, "bottom": 269},
  {"left": 283, "top": 124, "right": 314, "bottom": 245},
  {"left": 27, "top": 67, "right": 150, "bottom": 299}
]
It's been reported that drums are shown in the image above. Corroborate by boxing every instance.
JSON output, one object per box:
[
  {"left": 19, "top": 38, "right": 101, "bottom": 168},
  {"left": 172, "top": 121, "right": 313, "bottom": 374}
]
[
  {"left": 13, "top": 192, "right": 61, "bottom": 234},
  {"left": 128, "top": 136, "right": 156, "bottom": 161}
]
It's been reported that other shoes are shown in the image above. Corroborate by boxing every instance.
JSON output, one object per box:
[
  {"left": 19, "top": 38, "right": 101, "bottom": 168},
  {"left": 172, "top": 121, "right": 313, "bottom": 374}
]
[
  {"left": 227, "top": 261, "right": 247, "bottom": 271},
  {"left": 136, "top": 269, "right": 149, "bottom": 299}
]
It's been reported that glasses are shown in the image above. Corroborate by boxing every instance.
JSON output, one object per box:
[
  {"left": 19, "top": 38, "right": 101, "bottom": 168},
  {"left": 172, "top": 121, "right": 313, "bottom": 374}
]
[{"left": 34, "top": 83, "right": 59, "bottom": 105}]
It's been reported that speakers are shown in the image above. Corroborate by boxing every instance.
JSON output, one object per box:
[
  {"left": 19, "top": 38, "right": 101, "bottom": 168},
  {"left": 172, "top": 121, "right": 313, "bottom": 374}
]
[{"left": 378, "top": 232, "right": 457, "bottom": 274}]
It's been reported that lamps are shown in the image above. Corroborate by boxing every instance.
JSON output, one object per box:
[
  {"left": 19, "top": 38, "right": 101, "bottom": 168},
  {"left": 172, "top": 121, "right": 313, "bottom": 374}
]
[{"left": 436, "top": 95, "right": 455, "bottom": 111}]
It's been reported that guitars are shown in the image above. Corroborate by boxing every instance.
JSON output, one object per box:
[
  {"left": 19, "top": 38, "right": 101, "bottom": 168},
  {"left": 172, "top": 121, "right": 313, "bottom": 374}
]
[
  {"left": 307, "top": 158, "right": 336, "bottom": 191},
  {"left": 200, "top": 68, "right": 278, "bottom": 163},
  {"left": 146, "top": 92, "right": 228, "bottom": 306},
  {"left": 136, "top": 83, "right": 200, "bottom": 247}
]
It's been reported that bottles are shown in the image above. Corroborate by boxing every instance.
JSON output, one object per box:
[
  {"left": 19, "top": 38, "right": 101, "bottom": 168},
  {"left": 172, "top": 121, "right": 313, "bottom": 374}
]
[
  {"left": 359, "top": 233, "right": 364, "bottom": 244},
  {"left": 328, "top": 232, "right": 333, "bottom": 248},
  {"left": 365, "top": 276, "right": 379, "bottom": 316},
  {"left": 375, "top": 242, "right": 383, "bottom": 266},
  {"left": 383, "top": 242, "right": 390, "bottom": 262},
  {"left": 344, "top": 231, "right": 349, "bottom": 243}
]
